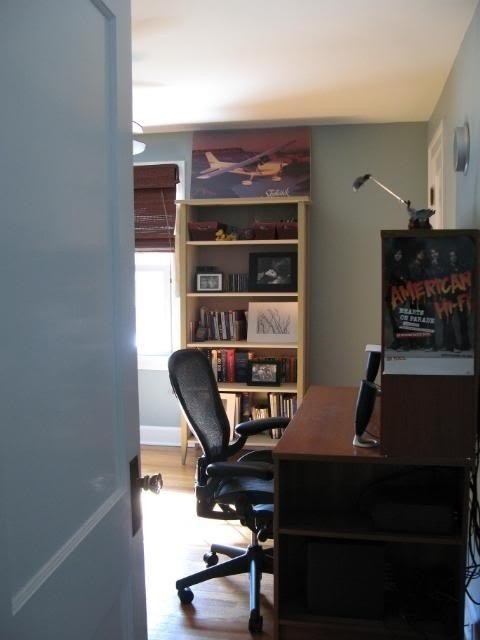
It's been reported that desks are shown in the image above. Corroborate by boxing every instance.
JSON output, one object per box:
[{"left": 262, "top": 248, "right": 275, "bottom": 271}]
[{"left": 273, "top": 384, "right": 470, "bottom": 640}]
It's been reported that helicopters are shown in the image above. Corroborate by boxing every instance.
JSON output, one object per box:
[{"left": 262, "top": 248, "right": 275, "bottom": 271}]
[{"left": 197, "top": 139, "right": 298, "bottom": 185}]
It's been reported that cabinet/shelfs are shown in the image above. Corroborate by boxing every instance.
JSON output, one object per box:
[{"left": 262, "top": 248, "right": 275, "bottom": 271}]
[
  {"left": 379, "top": 228, "right": 480, "bottom": 447},
  {"left": 173, "top": 195, "right": 313, "bottom": 465}
]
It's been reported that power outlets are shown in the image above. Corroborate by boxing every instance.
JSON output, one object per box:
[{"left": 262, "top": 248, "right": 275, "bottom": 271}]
[{"left": 464, "top": 608, "right": 473, "bottom": 640}]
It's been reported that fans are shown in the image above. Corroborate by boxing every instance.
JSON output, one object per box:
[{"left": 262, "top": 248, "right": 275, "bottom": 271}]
[{"left": 132, "top": 121, "right": 146, "bottom": 155}]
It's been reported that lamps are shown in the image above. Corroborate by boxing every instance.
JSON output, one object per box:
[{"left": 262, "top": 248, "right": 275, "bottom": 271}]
[{"left": 352, "top": 174, "right": 408, "bottom": 207}]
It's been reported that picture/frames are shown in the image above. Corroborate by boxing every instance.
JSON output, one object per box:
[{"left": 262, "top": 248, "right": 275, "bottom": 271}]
[
  {"left": 247, "top": 359, "right": 281, "bottom": 387},
  {"left": 247, "top": 301, "right": 298, "bottom": 343},
  {"left": 249, "top": 252, "right": 297, "bottom": 292},
  {"left": 196, "top": 273, "right": 223, "bottom": 291},
  {"left": 219, "top": 393, "right": 236, "bottom": 441}
]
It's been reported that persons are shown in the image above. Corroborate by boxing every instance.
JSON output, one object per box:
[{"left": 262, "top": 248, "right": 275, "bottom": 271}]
[{"left": 388, "top": 246, "right": 478, "bottom": 352}]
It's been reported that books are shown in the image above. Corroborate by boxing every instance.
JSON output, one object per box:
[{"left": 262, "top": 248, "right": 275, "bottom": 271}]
[
  {"left": 235, "top": 394, "right": 295, "bottom": 439},
  {"left": 200, "top": 349, "right": 295, "bottom": 382},
  {"left": 198, "top": 307, "right": 245, "bottom": 340}
]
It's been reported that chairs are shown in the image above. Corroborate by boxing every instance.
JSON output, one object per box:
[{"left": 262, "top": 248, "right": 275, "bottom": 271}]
[{"left": 167, "top": 349, "right": 292, "bottom": 634}]
